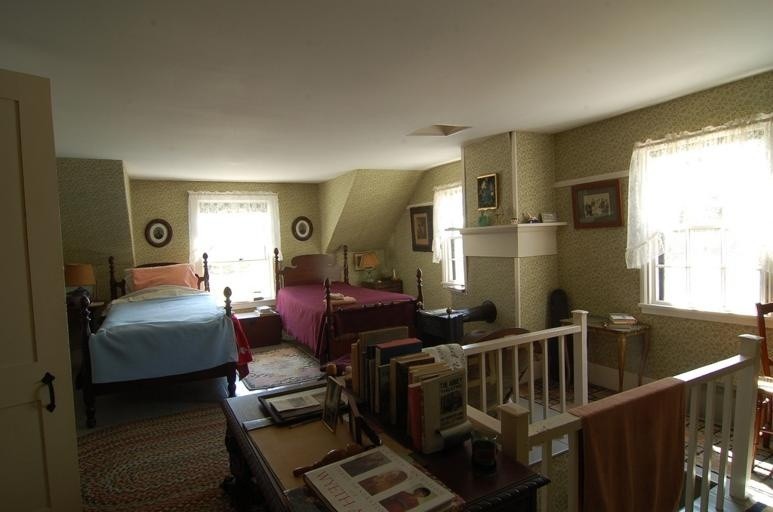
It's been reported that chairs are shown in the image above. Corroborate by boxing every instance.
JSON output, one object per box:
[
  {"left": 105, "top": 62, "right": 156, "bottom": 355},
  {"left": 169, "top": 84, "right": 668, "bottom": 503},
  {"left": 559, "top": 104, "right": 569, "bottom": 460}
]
[
  {"left": 66, "top": 290, "right": 92, "bottom": 350},
  {"left": 745, "top": 300, "right": 773, "bottom": 455}
]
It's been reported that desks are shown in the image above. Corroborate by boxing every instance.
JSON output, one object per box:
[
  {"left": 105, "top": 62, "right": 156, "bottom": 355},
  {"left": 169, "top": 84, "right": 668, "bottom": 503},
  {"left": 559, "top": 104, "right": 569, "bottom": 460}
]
[
  {"left": 556, "top": 312, "right": 653, "bottom": 395},
  {"left": 219, "top": 377, "right": 550, "bottom": 511}
]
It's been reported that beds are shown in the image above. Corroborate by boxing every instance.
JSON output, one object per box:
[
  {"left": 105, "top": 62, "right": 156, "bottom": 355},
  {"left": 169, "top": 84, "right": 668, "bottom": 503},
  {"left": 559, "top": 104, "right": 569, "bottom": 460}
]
[
  {"left": 83, "top": 253, "right": 251, "bottom": 426},
  {"left": 273, "top": 245, "right": 424, "bottom": 370}
]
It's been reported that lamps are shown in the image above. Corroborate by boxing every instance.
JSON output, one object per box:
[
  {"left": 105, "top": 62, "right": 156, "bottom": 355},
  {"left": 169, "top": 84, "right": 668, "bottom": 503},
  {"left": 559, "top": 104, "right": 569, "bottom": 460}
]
[
  {"left": 62, "top": 262, "right": 97, "bottom": 288},
  {"left": 358, "top": 251, "right": 380, "bottom": 282}
]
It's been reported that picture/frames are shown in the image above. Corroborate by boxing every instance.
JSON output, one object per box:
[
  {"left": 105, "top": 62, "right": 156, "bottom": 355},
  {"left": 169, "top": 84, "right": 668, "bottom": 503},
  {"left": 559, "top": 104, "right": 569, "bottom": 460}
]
[
  {"left": 476, "top": 172, "right": 499, "bottom": 211},
  {"left": 321, "top": 375, "right": 343, "bottom": 435},
  {"left": 409, "top": 206, "right": 433, "bottom": 252},
  {"left": 569, "top": 177, "right": 625, "bottom": 230}
]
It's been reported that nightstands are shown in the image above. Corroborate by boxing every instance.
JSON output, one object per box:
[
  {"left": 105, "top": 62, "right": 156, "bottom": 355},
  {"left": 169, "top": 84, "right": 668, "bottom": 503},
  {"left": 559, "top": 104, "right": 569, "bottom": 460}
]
[
  {"left": 360, "top": 279, "right": 403, "bottom": 295},
  {"left": 234, "top": 306, "right": 282, "bottom": 349}
]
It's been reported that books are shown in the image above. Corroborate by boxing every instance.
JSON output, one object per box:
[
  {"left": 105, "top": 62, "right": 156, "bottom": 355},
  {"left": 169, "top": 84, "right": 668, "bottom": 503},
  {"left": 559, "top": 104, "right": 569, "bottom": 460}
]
[
  {"left": 268, "top": 393, "right": 320, "bottom": 418},
  {"left": 606, "top": 321, "right": 630, "bottom": 329},
  {"left": 608, "top": 312, "right": 637, "bottom": 325},
  {"left": 253, "top": 310, "right": 279, "bottom": 317},
  {"left": 256, "top": 305, "right": 271, "bottom": 313},
  {"left": 303, "top": 445, "right": 456, "bottom": 511},
  {"left": 349, "top": 324, "right": 467, "bottom": 456},
  {"left": 283, "top": 484, "right": 330, "bottom": 512}
]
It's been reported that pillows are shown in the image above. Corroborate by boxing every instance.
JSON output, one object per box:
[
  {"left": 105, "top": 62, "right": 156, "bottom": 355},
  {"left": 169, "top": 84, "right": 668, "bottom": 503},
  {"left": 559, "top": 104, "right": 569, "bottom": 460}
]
[{"left": 122, "top": 261, "right": 200, "bottom": 294}]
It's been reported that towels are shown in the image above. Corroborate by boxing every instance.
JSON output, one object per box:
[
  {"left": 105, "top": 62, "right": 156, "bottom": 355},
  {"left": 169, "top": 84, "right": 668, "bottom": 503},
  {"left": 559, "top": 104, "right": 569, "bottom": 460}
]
[{"left": 567, "top": 376, "right": 686, "bottom": 512}]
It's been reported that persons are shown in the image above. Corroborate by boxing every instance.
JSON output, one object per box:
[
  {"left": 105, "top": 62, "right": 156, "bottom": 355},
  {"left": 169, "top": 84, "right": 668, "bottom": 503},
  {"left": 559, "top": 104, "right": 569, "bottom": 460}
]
[
  {"left": 359, "top": 470, "right": 408, "bottom": 495},
  {"left": 381, "top": 485, "right": 430, "bottom": 511}
]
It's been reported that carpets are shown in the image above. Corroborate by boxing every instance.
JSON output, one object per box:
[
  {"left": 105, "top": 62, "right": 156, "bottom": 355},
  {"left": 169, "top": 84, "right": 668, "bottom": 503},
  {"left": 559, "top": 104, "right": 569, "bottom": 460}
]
[
  {"left": 235, "top": 342, "right": 327, "bottom": 390},
  {"left": 74, "top": 406, "right": 232, "bottom": 512}
]
